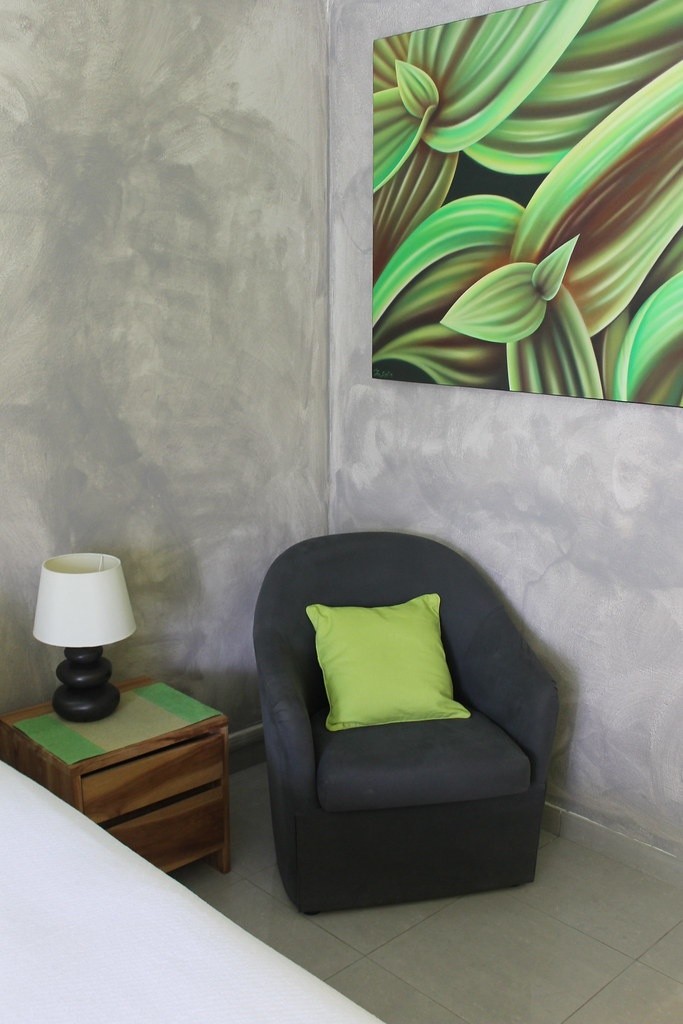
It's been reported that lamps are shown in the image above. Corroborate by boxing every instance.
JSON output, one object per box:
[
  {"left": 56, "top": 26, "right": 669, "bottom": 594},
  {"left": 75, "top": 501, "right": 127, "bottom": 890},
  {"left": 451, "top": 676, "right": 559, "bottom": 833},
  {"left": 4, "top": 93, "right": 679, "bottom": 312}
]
[{"left": 33, "top": 552, "right": 137, "bottom": 724}]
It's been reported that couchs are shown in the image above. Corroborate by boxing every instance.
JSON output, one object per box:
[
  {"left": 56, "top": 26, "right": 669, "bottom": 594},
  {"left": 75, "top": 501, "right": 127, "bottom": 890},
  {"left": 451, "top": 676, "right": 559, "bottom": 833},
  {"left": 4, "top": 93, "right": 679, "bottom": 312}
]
[{"left": 254, "top": 532, "right": 562, "bottom": 919}]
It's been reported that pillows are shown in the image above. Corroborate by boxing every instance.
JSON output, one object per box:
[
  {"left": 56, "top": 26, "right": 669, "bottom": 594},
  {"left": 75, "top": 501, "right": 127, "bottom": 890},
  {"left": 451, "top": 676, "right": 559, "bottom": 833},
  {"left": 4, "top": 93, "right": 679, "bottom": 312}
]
[{"left": 307, "top": 593, "right": 472, "bottom": 732}]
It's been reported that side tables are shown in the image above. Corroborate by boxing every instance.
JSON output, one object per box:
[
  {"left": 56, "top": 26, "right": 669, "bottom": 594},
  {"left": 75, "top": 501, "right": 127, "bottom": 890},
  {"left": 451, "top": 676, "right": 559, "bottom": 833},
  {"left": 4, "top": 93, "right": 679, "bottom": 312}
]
[{"left": 0, "top": 676, "right": 232, "bottom": 873}]
